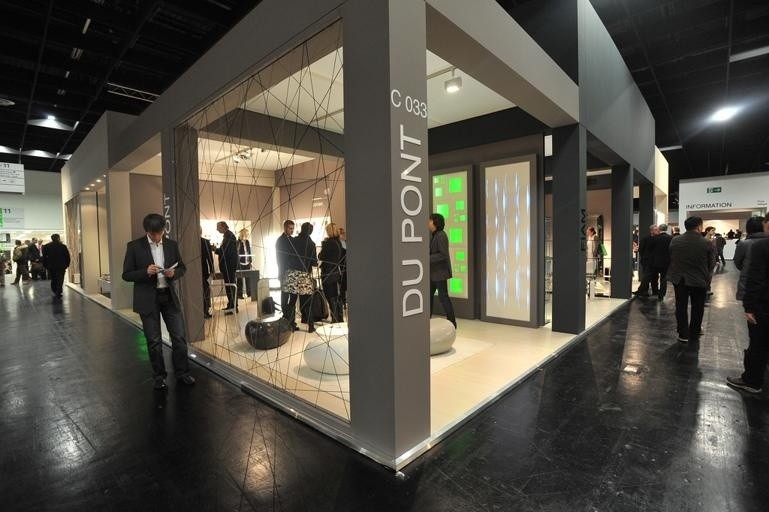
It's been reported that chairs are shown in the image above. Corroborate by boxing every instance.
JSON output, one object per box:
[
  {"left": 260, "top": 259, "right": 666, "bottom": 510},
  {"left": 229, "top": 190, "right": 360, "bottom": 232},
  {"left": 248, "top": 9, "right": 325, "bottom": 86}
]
[
  {"left": 256, "top": 277, "right": 276, "bottom": 317},
  {"left": 207, "top": 283, "right": 242, "bottom": 340}
]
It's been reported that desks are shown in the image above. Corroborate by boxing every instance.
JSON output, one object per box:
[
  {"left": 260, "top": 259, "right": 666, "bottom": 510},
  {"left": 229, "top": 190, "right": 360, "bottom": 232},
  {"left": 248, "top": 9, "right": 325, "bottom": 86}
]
[{"left": 213, "top": 269, "right": 259, "bottom": 301}]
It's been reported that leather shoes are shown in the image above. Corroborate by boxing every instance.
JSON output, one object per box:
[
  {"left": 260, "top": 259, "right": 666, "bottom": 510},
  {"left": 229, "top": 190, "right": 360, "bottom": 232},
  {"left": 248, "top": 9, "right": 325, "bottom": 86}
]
[
  {"left": 652, "top": 290, "right": 663, "bottom": 302},
  {"left": 632, "top": 290, "right": 650, "bottom": 297},
  {"left": 151, "top": 378, "right": 168, "bottom": 391},
  {"left": 175, "top": 370, "right": 196, "bottom": 386},
  {"left": 222, "top": 304, "right": 239, "bottom": 315},
  {"left": 9, "top": 277, "right": 41, "bottom": 285}
]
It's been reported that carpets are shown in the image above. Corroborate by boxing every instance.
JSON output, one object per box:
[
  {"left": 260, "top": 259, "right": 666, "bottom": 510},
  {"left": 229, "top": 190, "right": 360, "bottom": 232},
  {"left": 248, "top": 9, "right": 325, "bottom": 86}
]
[{"left": 215, "top": 316, "right": 493, "bottom": 402}]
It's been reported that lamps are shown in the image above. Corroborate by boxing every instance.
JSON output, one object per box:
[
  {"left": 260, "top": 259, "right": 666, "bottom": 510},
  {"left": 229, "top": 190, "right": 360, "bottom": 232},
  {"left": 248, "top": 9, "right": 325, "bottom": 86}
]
[
  {"left": 445, "top": 69, "right": 462, "bottom": 93},
  {"left": 232, "top": 152, "right": 251, "bottom": 163}
]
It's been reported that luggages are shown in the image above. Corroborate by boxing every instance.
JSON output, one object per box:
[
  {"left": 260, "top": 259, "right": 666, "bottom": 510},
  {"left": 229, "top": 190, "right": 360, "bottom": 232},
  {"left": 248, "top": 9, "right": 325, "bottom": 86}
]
[{"left": 313, "top": 289, "right": 329, "bottom": 321}]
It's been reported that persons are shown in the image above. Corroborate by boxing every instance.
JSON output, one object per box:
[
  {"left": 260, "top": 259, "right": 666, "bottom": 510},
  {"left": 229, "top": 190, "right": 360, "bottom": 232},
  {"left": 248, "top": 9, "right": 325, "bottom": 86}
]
[
  {"left": 281, "top": 221, "right": 318, "bottom": 333},
  {"left": 336, "top": 227, "right": 346, "bottom": 312},
  {"left": 276, "top": 219, "right": 300, "bottom": 331},
  {"left": 429, "top": 212, "right": 457, "bottom": 328},
  {"left": 40, "top": 234, "right": 71, "bottom": 299},
  {"left": 211, "top": 222, "right": 240, "bottom": 315},
  {"left": 122, "top": 214, "right": 196, "bottom": 390},
  {"left": 317, "top": 223, "right": 343, "bottom": 324},
  {"left": 199, "top": 223, "right": 215, "bottom": 319},
  {"left": 586, "top": 225, "right": 600, "bottom": 283},
  {"left": 0, "top": 229, "right": 51, "bottom": 286},
  {"left": 236, "top": 227, "right": 252, "bottom": 300},
  {"left": 631, "top": 208, "right": 768, "bottom": 395}
]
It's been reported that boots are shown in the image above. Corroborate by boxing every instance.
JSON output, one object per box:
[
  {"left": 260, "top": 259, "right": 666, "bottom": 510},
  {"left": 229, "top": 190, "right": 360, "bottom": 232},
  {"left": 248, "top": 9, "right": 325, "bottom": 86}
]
[{"left": 308, "top": 322, "right": 316, "bottom": 334}]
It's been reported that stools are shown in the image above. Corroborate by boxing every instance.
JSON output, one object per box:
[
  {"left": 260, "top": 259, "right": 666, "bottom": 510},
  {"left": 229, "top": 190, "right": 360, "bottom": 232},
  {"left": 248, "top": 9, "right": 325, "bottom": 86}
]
[
  {"left": 429, "top": 317, "right": 456, "bottom": 355},
  {"left": 304, "top": 324, "right": 349, "bottom": 374},
  {"left": 245, "top": 311, "right": 291, "bottom": 349}
]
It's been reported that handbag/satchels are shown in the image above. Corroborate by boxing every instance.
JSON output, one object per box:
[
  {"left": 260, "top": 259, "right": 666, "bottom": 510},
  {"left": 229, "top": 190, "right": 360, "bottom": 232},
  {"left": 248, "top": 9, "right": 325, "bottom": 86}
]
[
  {"left": 262, "top": 297, "right": 277, "bottom": 314},
  {"left": 598, "top": 244, "right": 607, "bottom": 256},
  {"left": 282, "top": 269, "right": 315, "bottom": 296},
  {"left": 31, "top": 261, "right": 43, "bottom": 273}
]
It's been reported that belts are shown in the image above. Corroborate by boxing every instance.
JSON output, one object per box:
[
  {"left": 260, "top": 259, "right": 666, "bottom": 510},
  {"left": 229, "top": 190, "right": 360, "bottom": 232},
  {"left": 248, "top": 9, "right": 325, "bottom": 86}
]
[{"left": 157, "top": 286, "right": 170, "bottom": 295}]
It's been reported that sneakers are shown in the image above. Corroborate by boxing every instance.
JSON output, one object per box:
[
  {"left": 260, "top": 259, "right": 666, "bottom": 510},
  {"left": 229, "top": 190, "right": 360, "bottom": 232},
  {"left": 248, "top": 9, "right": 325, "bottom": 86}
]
[
  {"left": 705, "top": 289, "right": 714, "bottom": 307},
  {"left": 690, "top": 326, "right": 704, "bottom": 334},
  {"left": 726, "top": 374, "right": 763, "bottom": 394},
  {"left": 677, "top": 333, "right": 689, "bottom": 342}
]
